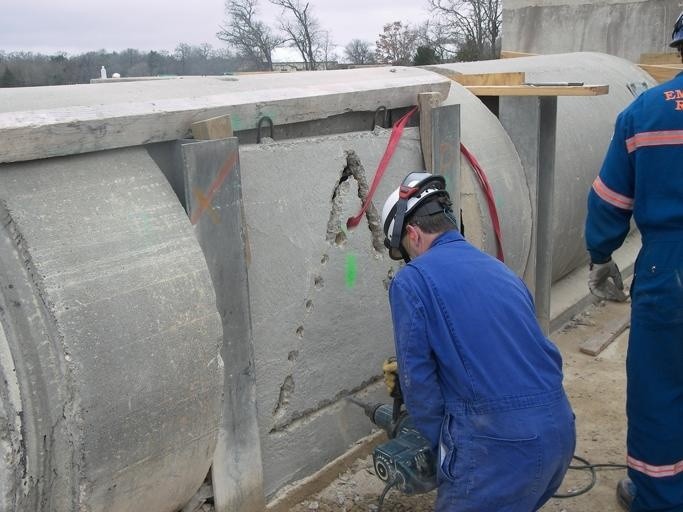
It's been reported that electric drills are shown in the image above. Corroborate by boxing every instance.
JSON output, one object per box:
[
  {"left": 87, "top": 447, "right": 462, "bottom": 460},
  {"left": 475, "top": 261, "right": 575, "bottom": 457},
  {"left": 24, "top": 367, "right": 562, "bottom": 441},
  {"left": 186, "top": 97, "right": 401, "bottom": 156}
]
[{"left": 345, "top": 355, "right": 439, "bottom": 498}]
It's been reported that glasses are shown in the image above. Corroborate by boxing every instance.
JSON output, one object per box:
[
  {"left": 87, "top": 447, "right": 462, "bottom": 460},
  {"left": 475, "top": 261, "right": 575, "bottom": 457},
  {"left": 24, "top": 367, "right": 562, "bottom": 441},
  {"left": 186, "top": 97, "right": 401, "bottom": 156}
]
[{"left": 392, "top": 172, "right": 444, "bottom": 253}]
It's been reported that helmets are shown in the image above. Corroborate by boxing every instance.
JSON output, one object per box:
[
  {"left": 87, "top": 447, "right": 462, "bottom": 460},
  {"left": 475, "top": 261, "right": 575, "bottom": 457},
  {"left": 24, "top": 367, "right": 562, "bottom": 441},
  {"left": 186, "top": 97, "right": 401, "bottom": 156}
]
[
  {"left": 382, "top": 170, "right": 452, "bottom": 260},
  {"left": 669, "top": 12, "right": 683, "bottom": 47}
]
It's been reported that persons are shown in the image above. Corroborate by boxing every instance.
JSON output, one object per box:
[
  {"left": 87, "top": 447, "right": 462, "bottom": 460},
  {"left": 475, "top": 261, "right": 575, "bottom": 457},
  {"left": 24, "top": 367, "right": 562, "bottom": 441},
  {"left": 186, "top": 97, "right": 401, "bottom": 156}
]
[
  {"left": 585, "top": 12, "right": 683, "bottom": 512},
  {"left": 381, "top": 171, "right": 576, "bottom": 512}
]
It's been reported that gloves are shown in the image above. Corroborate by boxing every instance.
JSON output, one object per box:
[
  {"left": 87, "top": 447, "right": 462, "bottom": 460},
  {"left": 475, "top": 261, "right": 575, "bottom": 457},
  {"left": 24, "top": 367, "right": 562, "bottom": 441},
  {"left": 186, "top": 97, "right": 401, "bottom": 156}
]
[
  {"left": 589, "top": 258, "right": 627, "bottom": 303},
  {"left": 383, "top": 360, "right": 399, "bottom": 390}
]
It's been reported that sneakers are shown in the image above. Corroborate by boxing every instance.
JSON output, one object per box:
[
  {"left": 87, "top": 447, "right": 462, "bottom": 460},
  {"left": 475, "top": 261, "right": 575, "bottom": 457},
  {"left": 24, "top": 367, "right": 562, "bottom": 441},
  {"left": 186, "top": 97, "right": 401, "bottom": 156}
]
[{"left": 616, "top": 479, "right": 637, "bottom": 509}]
